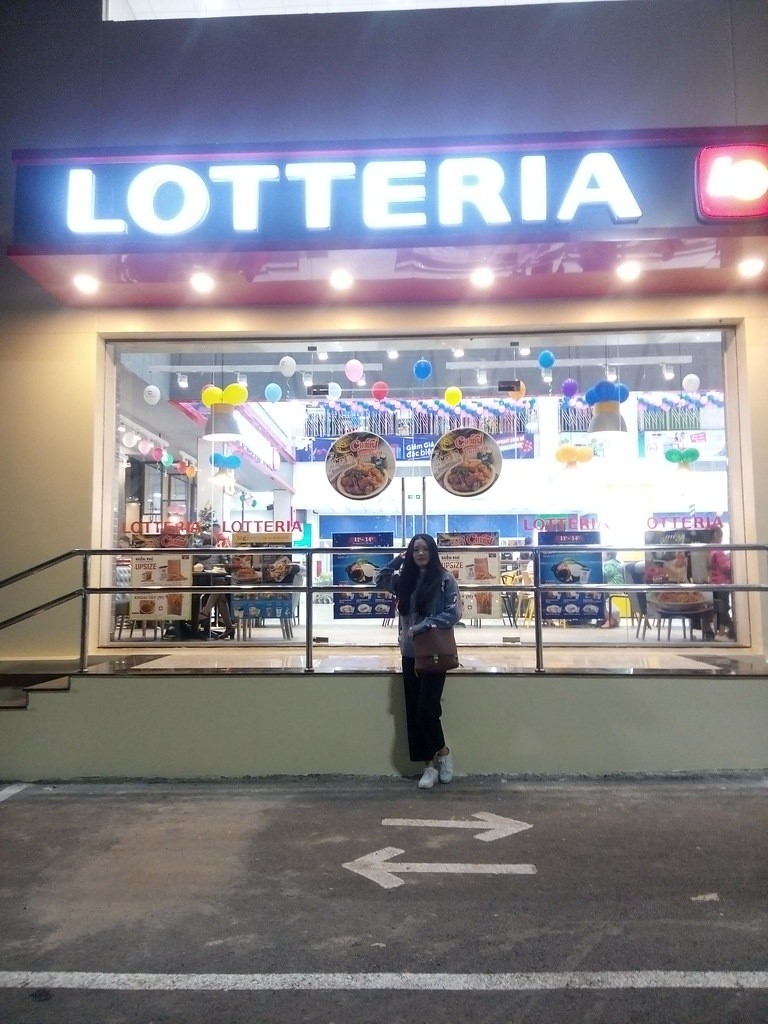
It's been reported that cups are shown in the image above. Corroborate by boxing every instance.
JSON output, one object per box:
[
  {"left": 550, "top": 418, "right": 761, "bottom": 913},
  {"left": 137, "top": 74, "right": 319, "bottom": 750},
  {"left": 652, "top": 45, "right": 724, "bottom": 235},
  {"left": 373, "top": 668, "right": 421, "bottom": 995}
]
[
  {"left": 580, "top": 567, "right": 591, "bottom": 584},
  {"left": 194, "top": 565, "right": 203, "bottom": 571}
]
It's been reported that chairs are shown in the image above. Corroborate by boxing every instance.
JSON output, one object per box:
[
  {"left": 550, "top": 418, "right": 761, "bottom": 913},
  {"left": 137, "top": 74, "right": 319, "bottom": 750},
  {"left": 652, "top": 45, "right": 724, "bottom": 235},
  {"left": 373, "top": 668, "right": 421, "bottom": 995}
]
[
  {"left": 617, "top": 563, "right": 726, "bottom": 644},
  {"left": 607, "top": 562, "right": 635, "bottom": 628},
  {"left": 501, "top": 571, "right": 534, "bottom": 627}
]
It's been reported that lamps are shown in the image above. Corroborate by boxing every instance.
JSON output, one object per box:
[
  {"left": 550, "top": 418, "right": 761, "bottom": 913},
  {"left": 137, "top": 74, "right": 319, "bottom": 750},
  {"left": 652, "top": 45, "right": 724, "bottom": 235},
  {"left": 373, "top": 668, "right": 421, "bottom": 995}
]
[
  {"left": 540, "top": 369, "right": 553, "bottom": 384},
  {"left": 478, "top": 369, "right": 487, "bottom": 386},
  {"left": 175, "top": 372, "right": 188, "bottom": 387},
  {"left": 236, "top": 372, "right": 247, "bottom": 387},
  {"left": 604, "top": 365, "right": 617, "bottom": 381},
  {"left": 385, "top": 350, "right": 400, "bottom": 360},
  {"left": 315, "top": 351, "right": 329, "bottom": 360},
  {"left": 453, "top": 348, "right": 464, "bottom": 357},
  {"left": 357, "top": 374, "right": 365, "bottom": 388},
  {"left": 302, "top": 372, "right": 313, "bottom": 387},
  {"left": 518, "top": 346, "right": 531, "bottom": 356},
  {"left": 202, "top": 403, "right": 241, "bottom": 441},
  {"left": 664, "top": 364, "right": 674, "bottom": 380},
  {"left": 587, "top": 401, "right": 628, "bottom": 435}
]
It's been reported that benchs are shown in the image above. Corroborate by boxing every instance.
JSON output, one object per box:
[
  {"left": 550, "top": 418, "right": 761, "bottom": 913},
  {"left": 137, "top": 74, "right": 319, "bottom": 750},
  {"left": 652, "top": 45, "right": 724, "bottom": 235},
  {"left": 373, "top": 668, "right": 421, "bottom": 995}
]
[
  {"left": 228, "top": 565, "right": 307, "bottom": 640},
  {"left": 115, "top": 558, "right": 163, "bottom": 640}
]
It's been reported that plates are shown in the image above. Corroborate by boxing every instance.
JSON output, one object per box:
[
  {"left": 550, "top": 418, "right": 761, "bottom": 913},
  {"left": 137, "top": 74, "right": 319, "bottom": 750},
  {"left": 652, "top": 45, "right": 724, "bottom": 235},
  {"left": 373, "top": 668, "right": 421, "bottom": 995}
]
[
  {"left": 337, "top": 463, "right": 388, "bottom": 499},
  {"left": 141, "top": 603, "right": 155, "bottom": 614},
  {"left": 444, "top": 458, "right": 496, "bottom": 496},
  {"left": 551, "top": 562, "right": 587, "bottom": 584},
  {"left": 345, "top": 560, "right": 379, "bottom": 584},
  {"left": 651, "top": 604, "right": 713, "bottom": 615},
  {"left": 204, "top": 570, "right": 222, "bottom": 573},
  {"left": 231, "top": 571, "right": 262, "bottom": 582}
]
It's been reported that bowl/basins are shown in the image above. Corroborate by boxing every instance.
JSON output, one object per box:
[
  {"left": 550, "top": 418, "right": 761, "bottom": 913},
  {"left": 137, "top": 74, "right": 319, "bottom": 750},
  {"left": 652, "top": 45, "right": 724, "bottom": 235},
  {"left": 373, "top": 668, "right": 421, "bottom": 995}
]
[{"left": 646, "top": 592, "right": 710, "bottom": 611}]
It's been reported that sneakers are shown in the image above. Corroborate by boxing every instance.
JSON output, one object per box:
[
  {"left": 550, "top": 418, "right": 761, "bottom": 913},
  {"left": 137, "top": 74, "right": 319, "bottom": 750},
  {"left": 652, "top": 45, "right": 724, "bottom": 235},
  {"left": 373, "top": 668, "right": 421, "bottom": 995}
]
[
  {"left": 418, "top": 767, "right": 439, "bottom": 788},
  {"left": 436, "top": 746, "right": 453, "bottom": 783}
]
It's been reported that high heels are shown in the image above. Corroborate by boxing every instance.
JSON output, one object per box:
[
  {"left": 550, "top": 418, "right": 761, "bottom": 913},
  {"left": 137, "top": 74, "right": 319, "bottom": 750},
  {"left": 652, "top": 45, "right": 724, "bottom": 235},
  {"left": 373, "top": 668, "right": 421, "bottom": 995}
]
[
  {"left": 198, "top": 611, "right": 210, "bottom": 627},
  {"left": 218, "top": 627, "right": 235, "bottom": 640}
]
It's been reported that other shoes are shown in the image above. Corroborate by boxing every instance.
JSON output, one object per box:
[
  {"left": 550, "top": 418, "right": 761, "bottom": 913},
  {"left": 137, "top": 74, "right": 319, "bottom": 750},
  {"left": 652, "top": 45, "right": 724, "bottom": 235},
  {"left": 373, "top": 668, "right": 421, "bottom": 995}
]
[{"left": 601, "top": 617, "right": 619, "bottom": 628}]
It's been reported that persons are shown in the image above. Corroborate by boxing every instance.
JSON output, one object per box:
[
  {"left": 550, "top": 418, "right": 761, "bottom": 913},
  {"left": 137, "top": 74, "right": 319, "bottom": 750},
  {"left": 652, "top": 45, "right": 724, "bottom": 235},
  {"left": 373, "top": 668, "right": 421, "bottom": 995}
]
[
  {"left": 115, "top": 523, "right": 236, "bottom": 641},
  {"left": 711, "top": 526, "right": 737, "bottom": 640},
  {"left": 375, "top": 534, "right": 462, "bottom": 789},
  {"left": 597, "top": 553, "right": 624, "bottom": 630},
  {"left": 501, "top": 538, "right": 536, "bottom": 560}
]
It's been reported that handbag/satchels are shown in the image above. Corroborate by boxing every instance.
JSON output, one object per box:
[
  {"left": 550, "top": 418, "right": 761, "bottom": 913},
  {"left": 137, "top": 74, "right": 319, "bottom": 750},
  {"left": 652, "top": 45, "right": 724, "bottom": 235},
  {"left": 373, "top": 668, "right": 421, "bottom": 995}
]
[{"left": 412, "top": 627, "right": 459, "bottom": 677}]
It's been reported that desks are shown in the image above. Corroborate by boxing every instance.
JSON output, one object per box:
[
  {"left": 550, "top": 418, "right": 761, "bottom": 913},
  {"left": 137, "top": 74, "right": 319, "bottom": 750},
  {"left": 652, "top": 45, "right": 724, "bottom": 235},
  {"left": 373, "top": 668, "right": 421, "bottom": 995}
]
[{"left": 176, "top": 571, "right": 227, "bottom": 638}]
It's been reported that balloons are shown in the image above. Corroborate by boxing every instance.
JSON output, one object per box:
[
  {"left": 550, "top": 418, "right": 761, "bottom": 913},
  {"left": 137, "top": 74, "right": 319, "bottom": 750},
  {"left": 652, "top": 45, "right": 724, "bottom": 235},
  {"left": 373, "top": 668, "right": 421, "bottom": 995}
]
[{"left": 122, "top": 351, "right": 701, "bottom": 516}]
